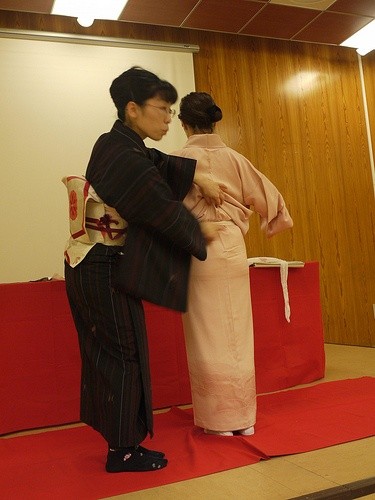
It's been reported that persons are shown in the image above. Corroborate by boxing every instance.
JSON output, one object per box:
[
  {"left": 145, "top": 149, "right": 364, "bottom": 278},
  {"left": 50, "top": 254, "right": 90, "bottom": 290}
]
[
  {"left": 167, "top": 93, "right": 295, "bottom": 437},
  {"left": 63, "top": 66, "right": 226, "bottom": 475}
]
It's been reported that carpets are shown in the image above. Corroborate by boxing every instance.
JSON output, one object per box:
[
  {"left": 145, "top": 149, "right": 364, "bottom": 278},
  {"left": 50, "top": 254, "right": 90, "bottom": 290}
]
[{"left": 2, "top": 370, "right": 375, "bottom": 498}]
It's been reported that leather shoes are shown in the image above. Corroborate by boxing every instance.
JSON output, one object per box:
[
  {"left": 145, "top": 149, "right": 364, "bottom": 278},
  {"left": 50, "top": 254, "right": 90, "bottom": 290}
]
[
  {"left": 128, "top": 446, "right": 164, "bottom": 458},
  {"left": 106, "top": 447, "right": 167, "bottom": 472}
]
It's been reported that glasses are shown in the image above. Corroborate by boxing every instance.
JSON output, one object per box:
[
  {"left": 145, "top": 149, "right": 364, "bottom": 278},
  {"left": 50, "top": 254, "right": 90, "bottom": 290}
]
[{"left": 139, "top": 104, "right": 177, "bottom": 118}]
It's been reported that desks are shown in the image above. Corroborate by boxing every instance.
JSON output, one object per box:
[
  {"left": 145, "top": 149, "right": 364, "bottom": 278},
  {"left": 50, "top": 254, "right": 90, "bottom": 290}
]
[{"left": 0, "top": 260, "right": 329, "bottom": 440}]
